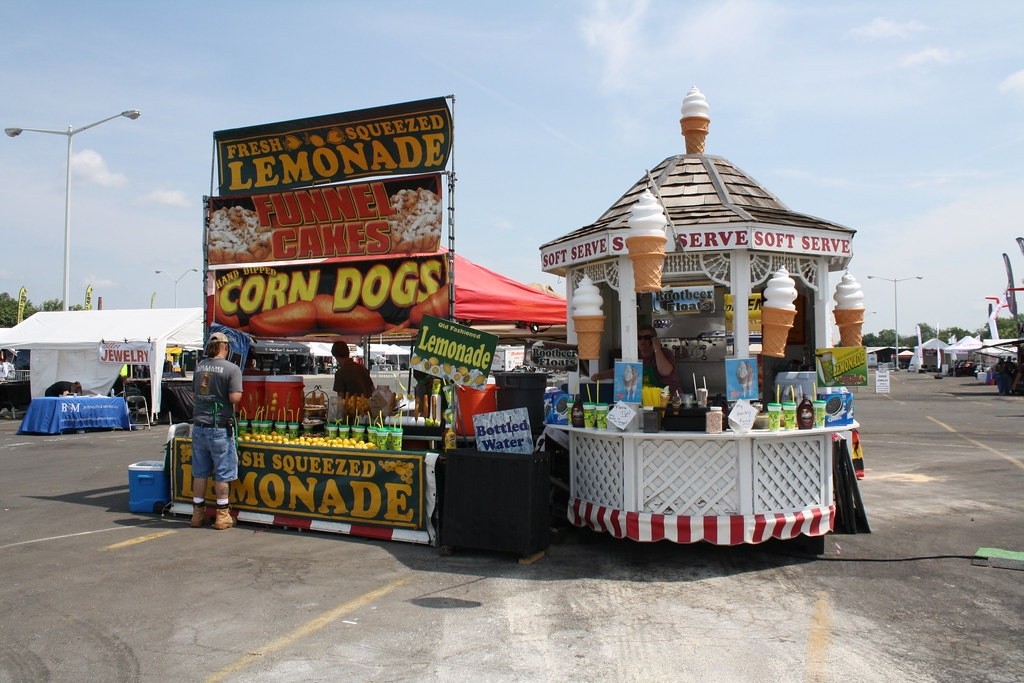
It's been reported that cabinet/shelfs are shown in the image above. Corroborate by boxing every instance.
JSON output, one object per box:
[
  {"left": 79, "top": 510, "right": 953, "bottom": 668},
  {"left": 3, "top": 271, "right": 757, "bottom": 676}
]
[{"left": 658, "top": 336, "right": 725, "bottom": 363}]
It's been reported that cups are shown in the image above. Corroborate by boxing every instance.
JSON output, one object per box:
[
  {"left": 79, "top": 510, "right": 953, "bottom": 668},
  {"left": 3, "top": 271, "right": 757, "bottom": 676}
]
[
  {"left": 582, "top": 402, "right": 607, "bottom": 430},
  {"left": 683, "top": 394, "right": 693, "bottom": 407},
  {"left": 768, "top": 403, "right": 797, "bottom": 430},
  {"left": 303, "top": 425, "right": 314, "bottom": 434},
  {"left": 624, "top": 402, "right": 639, "bottom": 432},
  {"left": 566, "top": 400, "right": 576, "bottom": 426},
  {"left": 812, "top": 401, "right": 826, "bottom": 428},
  {"left": 326, "top": 424, "right": 403, "bottom": 451},
  {"left": 696, "top": 388, "right": 709, "bottom": 408},
  {"left": 442, "top": 384, "right": 457, "bottom": 430}
]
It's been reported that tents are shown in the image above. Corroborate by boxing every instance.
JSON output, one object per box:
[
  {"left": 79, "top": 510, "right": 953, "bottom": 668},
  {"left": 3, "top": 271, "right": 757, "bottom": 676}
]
[
  {"left": 891, "top": 335, "right": 1024, "bottom": 380},
  {"left": 206, "top": 218, "right": 571, "bottom": 395},
  {"left": 0, "top": 307, "right": 410, "bottom": 427}
]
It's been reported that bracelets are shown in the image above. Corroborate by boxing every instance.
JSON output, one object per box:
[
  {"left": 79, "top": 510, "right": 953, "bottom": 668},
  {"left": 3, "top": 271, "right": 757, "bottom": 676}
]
[{"left": 653, "top": 348, "right": 663, "bottom": 353}]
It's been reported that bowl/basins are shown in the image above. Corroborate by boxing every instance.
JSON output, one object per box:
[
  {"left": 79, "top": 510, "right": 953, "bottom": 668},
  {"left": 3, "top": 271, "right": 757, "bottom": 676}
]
[
  {"left": 755, "top": 413, "right": 769, "bottom": 429},
  {"left": 727, "top": 417, "right": 750, "bottom": 434}
]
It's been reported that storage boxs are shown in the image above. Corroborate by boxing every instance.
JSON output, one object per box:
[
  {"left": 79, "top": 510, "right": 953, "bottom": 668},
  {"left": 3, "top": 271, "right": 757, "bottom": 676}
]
[
  {"left": 544, "top": 392, "right": 569, "bottom": 425},
  {"left": 817, "top": 392, "right": 854, "bottom": 427},
  {"left": 127, "top": 423, "right": 192, "bottom": 515}
]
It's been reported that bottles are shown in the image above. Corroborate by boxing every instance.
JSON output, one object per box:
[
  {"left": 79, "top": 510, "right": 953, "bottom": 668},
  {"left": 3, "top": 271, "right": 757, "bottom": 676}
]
[
  {"left": 609, "top": 404, "right": 618, "bottom": 431},
  {"left": 571, "top": 394, "right": 584, "bottom": 427},
  {"left": 369, "top": 385, "right": 392, "bottom": 417},
  {"left": 442, "top": 423, "right": 457, "bottom": 452},
  {"left": 797, "top": 394, "right": 814, "bottom": 429},
  {"left": 237, "top": 420, "right": 299, "bottom": 439},
  {"left": 706, "top": 406, "right": 723, "bottom": 434}
]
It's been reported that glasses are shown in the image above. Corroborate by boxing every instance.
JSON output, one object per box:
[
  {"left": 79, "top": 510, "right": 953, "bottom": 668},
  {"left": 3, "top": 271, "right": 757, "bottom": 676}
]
[{"left": 638, "top": 334, "right": 654, "bottom": 340}]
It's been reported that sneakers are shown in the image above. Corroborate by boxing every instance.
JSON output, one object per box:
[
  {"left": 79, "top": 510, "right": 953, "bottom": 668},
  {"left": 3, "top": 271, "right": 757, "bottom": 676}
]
[
  {"left": 214, "top": 508, "right": 237, "bottom": 529},
  {"left": 191, "top": 506, "right": 210, "bottom": 526}
]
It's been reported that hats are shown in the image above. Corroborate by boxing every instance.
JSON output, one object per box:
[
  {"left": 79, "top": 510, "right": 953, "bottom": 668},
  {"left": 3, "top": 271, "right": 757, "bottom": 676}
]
[{"left": 209, "top": 332, "right": 229, "bottom": 342}]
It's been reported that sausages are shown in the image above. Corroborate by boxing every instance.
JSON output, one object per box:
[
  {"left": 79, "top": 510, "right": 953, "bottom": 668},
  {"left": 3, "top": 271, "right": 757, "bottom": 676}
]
[
  {"left": 414, "top": 395, "right": 437, "bottom": 421},
  {"left": 248, "top": 390, "right": 292, "bottom": 414}
]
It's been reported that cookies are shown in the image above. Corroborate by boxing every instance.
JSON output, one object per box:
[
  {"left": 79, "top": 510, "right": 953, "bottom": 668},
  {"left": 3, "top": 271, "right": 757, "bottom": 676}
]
[
  {"left": 846, "top": 400, "right": 852, "bottom": 413},
  {"left": 545, "top": 404, "right": 552, "bottom": 417},
  {"left": 826, "top": 397, "right": 843, "bottom": 415},
  {"left": 556, "top": 397, "right": 568, "bottom": 414}
]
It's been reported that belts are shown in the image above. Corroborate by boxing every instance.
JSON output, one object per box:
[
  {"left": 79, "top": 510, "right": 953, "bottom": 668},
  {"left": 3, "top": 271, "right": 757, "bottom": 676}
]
[{"left": 195, "top": 422, "right": 227, "bottom": 428}]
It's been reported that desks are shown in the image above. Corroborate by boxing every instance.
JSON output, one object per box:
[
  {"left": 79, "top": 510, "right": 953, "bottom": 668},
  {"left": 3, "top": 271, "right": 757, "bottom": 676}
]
[{"left": 16, "top": 395, "right": 135, "bottom": 436}]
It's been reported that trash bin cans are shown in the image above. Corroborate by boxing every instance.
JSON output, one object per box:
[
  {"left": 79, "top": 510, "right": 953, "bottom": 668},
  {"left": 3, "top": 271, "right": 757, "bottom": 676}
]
[
  {"left": 128, "top": 460, "right": 170, "bottom": 513},
  {"left": 492, "top": 372, "right": 549, "bottom": 433},
  {"left": 977, "top": 372, "right": 987, "bottom": 384},
  {"left": 996, "top": 373, "right": 1010, "bottom": 394}
]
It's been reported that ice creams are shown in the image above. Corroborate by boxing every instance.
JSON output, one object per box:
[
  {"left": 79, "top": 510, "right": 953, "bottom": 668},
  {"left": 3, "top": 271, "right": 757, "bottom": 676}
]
[
  {"left": 623, "top": 364, "right": 638, "bottom": 394},
  {"left": 737, "top": 360, "right": 754, "bottom": 397},
  {"left": 679, "top": 85, "right": 710, "bottom": 155},
  {"left": 761, "top": 265, "right": 799, "bottom": 359},
  {"left": 571, "top": 273, "right": 605, "bottom": 359},
  {"left": 832, "top": 274, "right": 866, "bottom": 346},
  {"left": 624, "top": 188, "right": 668, "bottom": 293}
]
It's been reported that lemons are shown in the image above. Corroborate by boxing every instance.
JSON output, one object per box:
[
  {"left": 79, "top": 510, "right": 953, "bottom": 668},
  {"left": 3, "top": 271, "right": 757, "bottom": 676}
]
[
  {"left": 428, "top": 357, "right": 468, "bottom": 383},
  {"left": 236, "top": 432, "right": 377, "bottom": 449}
]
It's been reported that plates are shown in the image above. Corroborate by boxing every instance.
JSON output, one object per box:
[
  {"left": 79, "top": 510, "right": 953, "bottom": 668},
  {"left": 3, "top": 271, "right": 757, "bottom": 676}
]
[
  {"left": 302, "top": 423, "right": 325, "bottom": 425},
  {"left": 303, "top": 407, "right": 325, "bottom": 410}
]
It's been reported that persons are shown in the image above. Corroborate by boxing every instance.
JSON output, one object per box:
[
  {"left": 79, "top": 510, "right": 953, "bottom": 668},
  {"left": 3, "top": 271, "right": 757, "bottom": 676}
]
[
  {"left": 994, "top": 356, "right": 1024, "bottom": 395},
  {"left": 191, "top": 333, "right": 243, "bottom": 530},
  {"left": 413, "top": 366, "right": 448, "bottom": 413},
  {"left": 45, "top": 381, "right": 82, "bottom": 397},
  {"left": 331, "top": 340, "right": 375, "bottom": 417},
  {"left": 591, "top": 326, "right": 680, "bottom": 402}
]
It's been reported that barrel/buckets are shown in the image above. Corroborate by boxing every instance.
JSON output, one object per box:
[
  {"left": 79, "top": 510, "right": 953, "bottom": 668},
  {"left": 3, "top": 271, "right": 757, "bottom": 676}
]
[
  {"left": 235, "top": 376, "right": 265, "bottom": 420},
  {"left": 455, "top": 384, "right": 500, "bottom": 436},
  {"left": 265, "top": 376, "right": 304, "bottom": 421},
  {"left": 491, "top": 372, "right": 546, "bottom": 446}
]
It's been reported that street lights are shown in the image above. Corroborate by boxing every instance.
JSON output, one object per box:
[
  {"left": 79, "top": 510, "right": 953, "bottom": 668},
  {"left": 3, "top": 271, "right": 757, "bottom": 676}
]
[
  {"left": 4, "top": 109, "right": 142, "bottom": 311},
  {"left": 155, "top": 269, "right": 198, "bottom": 309},
  {"left": 868, "top": 275, "right": 923, "bottom": 371}
]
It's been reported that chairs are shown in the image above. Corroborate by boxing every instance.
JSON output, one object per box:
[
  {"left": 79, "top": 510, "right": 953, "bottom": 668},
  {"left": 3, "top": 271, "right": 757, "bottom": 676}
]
[{"left": 127, "top": 386, "right": 151, "bottom": 432}]
